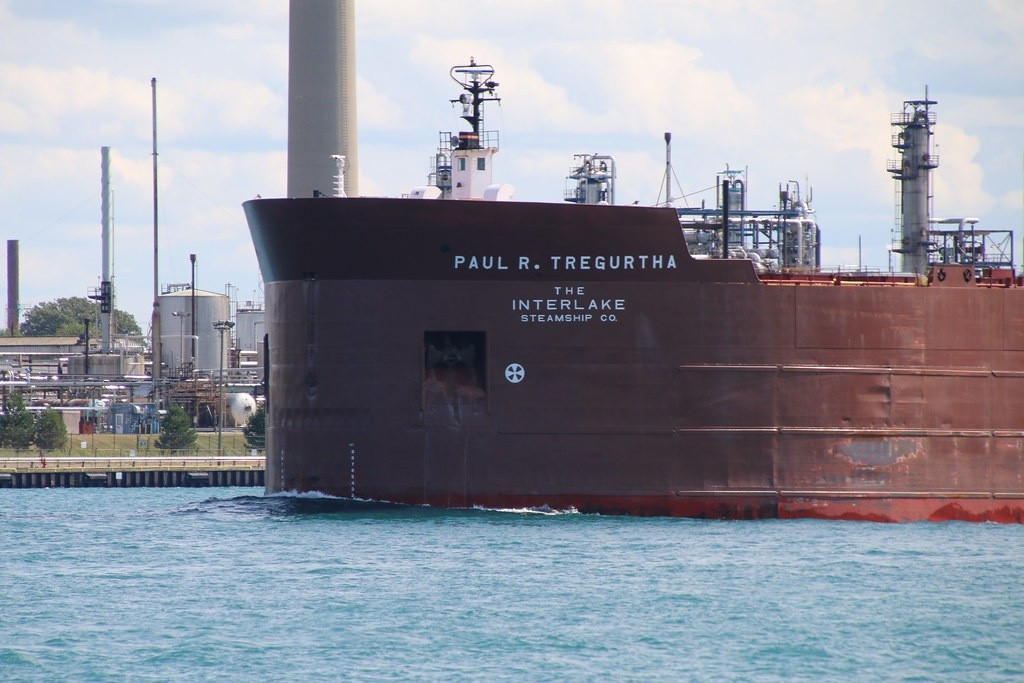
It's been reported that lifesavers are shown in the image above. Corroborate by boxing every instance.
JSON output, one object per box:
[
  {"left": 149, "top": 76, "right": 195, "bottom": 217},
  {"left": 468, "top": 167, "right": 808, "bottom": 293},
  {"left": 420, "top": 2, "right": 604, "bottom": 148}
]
[
  {"left": 937, "top": 268, "right": 946, "bottom": 281},
  {"left": 963, "top": 269, "right": 972, "bottom": 282}
]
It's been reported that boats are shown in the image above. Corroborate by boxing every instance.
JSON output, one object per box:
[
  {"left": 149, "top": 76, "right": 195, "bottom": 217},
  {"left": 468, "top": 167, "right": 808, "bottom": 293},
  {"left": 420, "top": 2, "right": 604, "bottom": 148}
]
[{"left": 240, "top": 58, "right": 1024, "bottom": 523}]
